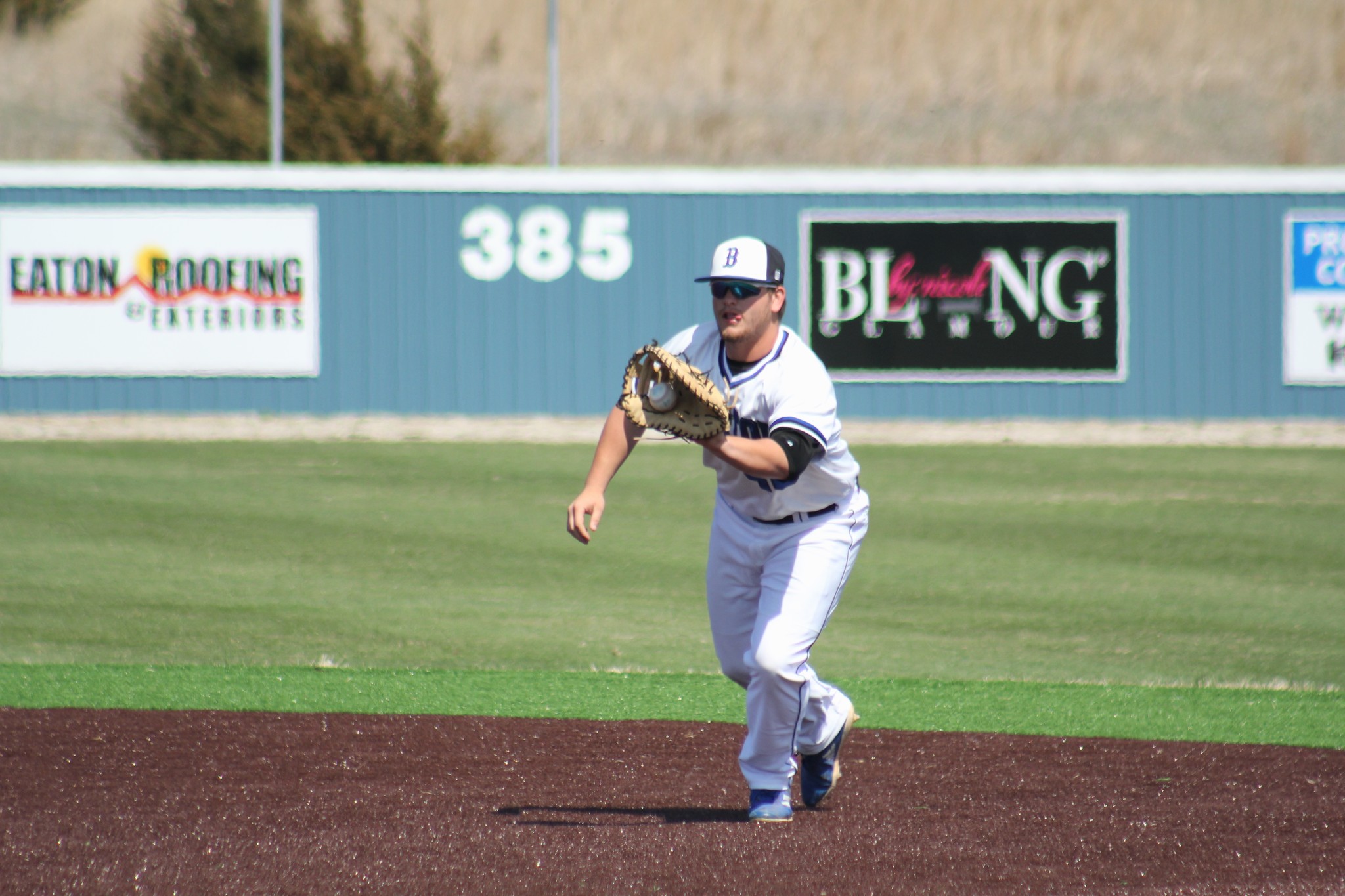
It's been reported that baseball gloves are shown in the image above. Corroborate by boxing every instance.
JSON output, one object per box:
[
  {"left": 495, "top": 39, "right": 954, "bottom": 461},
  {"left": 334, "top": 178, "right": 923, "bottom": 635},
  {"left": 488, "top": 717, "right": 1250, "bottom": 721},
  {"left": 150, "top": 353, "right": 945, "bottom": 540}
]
[{"left": 620, "top": 342, "right": 728, "bottom": 441}]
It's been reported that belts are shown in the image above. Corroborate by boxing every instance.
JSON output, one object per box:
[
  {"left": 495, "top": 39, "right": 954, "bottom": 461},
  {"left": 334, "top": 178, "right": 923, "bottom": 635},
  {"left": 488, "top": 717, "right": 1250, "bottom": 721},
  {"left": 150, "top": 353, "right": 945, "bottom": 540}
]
[{"left": 753, "top": 475, "right": 860, "bottom": 526}]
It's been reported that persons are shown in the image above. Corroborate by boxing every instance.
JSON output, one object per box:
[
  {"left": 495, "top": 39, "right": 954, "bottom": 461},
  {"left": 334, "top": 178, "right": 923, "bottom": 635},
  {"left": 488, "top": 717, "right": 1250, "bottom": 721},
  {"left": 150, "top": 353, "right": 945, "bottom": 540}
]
[{"left": 565, "top": 235, "right": 868, "bottom": 822}]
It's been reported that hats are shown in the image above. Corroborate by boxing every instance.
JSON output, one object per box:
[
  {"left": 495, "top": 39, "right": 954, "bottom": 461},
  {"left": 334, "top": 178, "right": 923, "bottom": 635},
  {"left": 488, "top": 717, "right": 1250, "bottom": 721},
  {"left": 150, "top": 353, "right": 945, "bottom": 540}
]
[{"left": 694, "top": 235, "right": 785, "bottom": 288}]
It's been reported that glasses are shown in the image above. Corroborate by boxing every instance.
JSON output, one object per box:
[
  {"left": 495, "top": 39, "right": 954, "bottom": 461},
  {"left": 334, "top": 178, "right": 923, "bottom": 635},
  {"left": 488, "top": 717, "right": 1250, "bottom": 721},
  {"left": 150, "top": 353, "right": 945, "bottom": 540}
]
[{"left": 710, "top": 282, "right": 761, "bottom": 299}]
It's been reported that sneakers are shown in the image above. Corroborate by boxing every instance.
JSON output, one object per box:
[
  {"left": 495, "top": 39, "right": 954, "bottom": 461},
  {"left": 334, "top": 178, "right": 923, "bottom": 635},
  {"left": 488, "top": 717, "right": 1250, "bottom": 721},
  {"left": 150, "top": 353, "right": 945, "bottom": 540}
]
[
  {"left": 748, "top": 789, "right": 793, "bottom": 823},
  {"left": 800, "top": 702, "right": 855, "bottom": 807}
]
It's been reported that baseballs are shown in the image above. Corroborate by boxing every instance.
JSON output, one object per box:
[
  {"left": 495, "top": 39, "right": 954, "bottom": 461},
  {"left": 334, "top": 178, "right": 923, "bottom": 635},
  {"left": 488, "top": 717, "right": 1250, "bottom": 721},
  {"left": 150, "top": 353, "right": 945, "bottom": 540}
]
[{"left": 649, "top": 382, "right": 677, "bottom": 411}]
